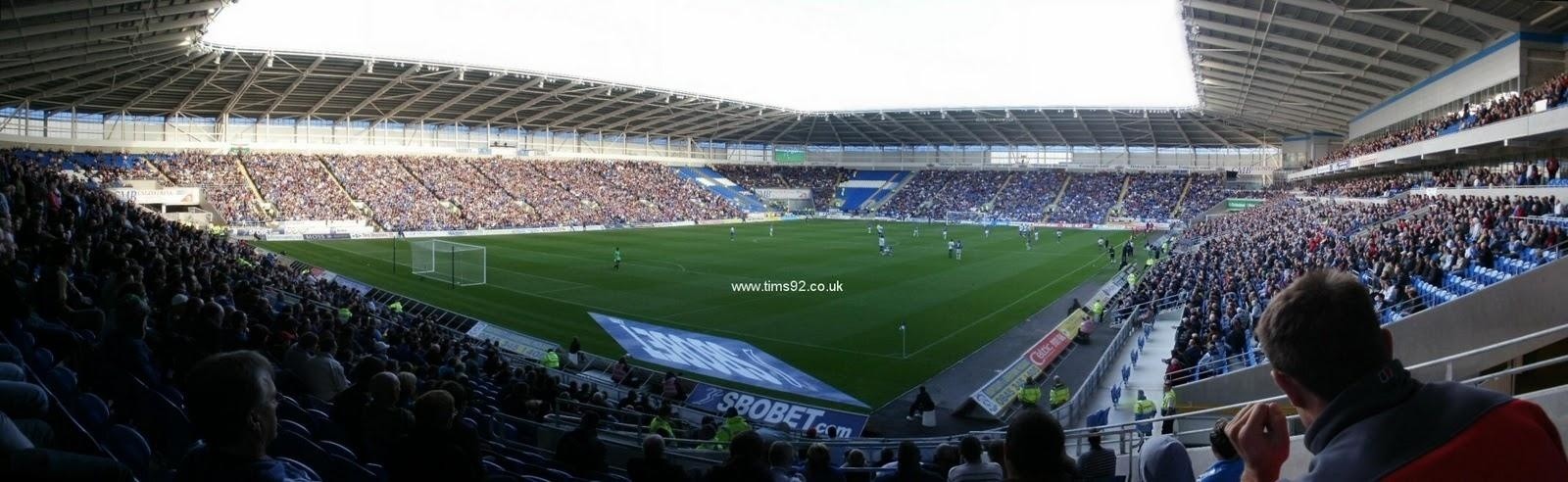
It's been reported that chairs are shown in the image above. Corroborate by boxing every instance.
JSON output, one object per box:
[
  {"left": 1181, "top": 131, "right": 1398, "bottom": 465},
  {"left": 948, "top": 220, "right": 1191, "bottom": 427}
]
[{"left": 0, "top": 107, "right": 1568, "bottom": 481}]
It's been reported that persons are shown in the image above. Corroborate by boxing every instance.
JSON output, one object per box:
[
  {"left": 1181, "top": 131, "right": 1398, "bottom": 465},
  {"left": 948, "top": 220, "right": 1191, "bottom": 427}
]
[{"left": 1, "top": 65, "right": 1567, "bottom": 481}]
